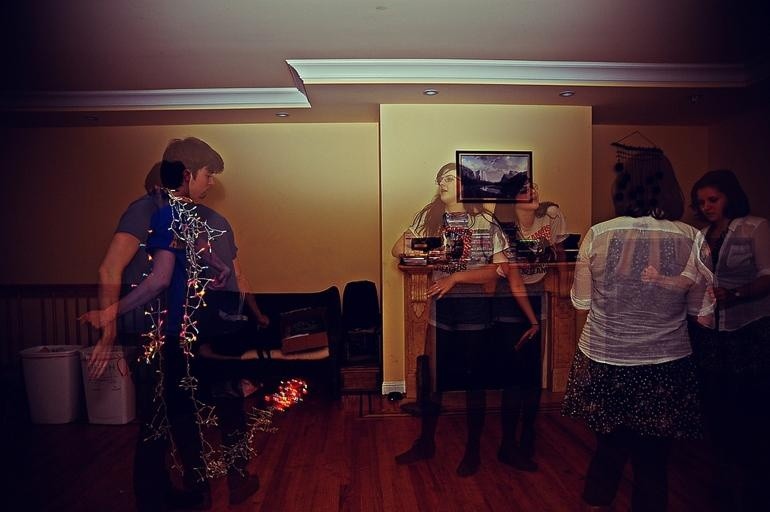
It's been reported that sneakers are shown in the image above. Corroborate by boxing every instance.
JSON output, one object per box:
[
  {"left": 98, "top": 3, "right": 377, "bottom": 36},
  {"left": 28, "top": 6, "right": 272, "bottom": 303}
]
[
  {"left": 500, "top": 450, "right": 540, "bottom": 473},
  {"left": 230, "top": 475, "right": 259, "bottom": 502},
  {"left": 457, "top": 453, "right": 478, "bottom": 476},
  {"left": 394, "top": 437, "right": 432, "bottom": 463}
]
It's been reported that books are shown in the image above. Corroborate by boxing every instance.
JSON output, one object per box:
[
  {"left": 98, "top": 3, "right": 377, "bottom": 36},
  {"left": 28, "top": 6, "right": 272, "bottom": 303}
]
[{"left": 398, "top": 212, "right": 581, "bottom": 268}]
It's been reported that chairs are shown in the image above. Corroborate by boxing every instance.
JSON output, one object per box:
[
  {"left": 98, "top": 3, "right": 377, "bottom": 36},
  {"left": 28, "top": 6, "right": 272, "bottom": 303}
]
[{"left": 343, "top": 280, "right": 382, "bottom": 367}]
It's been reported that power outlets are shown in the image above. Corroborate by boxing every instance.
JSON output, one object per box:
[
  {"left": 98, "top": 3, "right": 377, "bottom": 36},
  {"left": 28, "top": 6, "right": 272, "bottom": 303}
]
[{"left": 382, "top": 381, "right": 405, "bottom": 394}]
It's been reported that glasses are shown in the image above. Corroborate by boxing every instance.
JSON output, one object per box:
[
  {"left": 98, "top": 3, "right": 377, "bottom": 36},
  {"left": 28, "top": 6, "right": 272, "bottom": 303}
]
[{"left": 437, "top": 175, "right": 462, "bottom": 184}]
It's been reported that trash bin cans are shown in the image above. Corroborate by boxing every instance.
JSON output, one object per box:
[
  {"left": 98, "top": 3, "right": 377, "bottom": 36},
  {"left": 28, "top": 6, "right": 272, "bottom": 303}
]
[
  {"left": 21, "top": 344, "right": 84, "bottom": 424},
  {"left": 79, "top": 345, "right": 136, "bottom": 424}
]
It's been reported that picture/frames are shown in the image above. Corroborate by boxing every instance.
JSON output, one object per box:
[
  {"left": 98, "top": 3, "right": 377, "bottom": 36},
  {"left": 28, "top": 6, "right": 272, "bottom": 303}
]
[{"left": 456, "top": 150, "right": 533, "bottom": 203}]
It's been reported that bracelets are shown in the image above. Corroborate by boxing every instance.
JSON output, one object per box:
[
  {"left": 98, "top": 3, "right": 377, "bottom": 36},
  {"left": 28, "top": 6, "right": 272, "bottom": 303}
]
[{"left": 531, "top": 322, "right": 540, "bottom": 327}]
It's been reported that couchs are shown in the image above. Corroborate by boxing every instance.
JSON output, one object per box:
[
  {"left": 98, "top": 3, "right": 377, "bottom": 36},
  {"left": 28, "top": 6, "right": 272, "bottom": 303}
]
[{"left": 204, "top": 284, "right": 342, "bottom": 398}]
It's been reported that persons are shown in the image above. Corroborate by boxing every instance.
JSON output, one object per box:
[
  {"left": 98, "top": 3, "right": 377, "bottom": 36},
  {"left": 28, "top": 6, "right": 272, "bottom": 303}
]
[
  {"left": 390, "top": 162, "right": 513, "bottom": 477},
  {"left": 75, "top": 160, "right": 272, "bottom": 511},
  {"left": 489, "top": 172, "right": 572, "bottom": 474},
  {"left": 640, "top": 166, "right": 770, "bottom": 512},
  {"left": 560, "top": 149, "right": 726, "bottom": 512},
  {"left": 87, "top": 136, "right": 233, "bottom": 511}
]
